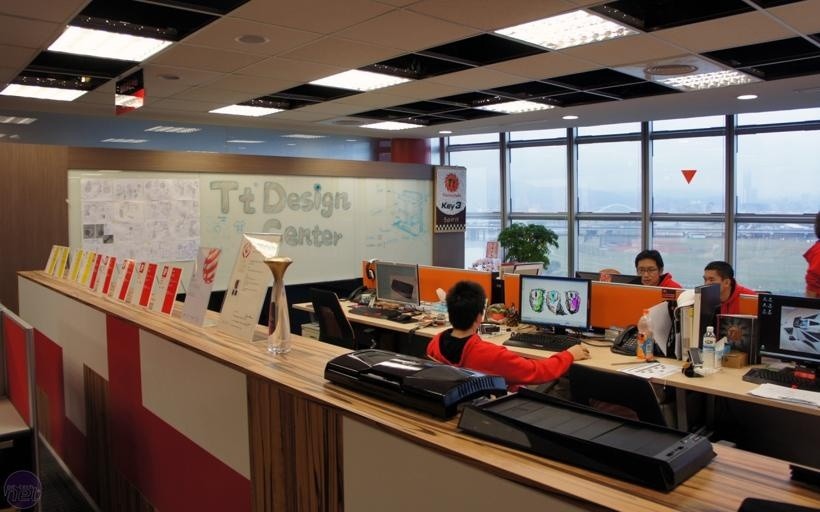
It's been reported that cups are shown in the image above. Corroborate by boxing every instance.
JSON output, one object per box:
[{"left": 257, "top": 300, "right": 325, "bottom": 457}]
[{"left": 506, "top": 312, "right": 519, "bottom": 328}]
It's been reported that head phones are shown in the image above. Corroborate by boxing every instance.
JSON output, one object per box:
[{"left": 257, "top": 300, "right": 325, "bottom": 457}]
[{"left": 366, "top": 258, "right": 378, "bottom": 280}]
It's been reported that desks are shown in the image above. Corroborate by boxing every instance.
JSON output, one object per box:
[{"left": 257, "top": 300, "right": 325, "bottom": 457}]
[
  {"left": 412, "top": 314, "right": 677, "bottom": 431},
  {"left": 292, "top": 298, "right": 424, "bottom": 357},
  {"left": 651, "top": 353, "right": 820, "bottom": 431}
]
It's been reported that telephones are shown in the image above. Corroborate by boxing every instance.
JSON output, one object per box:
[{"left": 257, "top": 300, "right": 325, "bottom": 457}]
[
  {"left": 610, "top": 324, "right": 639, "bottom": 356},
  {"left": 347, "top": 285, "right": 376, "bottom": 304}
]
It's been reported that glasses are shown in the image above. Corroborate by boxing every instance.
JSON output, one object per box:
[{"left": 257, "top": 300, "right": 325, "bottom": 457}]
[{"left": 637, "top": 266, "right": 658, "bottom": 275}]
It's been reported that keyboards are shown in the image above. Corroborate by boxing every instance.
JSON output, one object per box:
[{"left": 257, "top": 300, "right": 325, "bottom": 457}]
[
  {"left": 502, "top": 332, "right": 581, "bottom": 352},
  {"left": 351, "top": 307, "right": 401, "bottom": 319},
  {"left": 742, "top": 368, "right": 820, "bottom": 392}
]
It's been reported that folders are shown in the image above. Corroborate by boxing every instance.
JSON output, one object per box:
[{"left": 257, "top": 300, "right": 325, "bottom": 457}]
[{"left": 693, "top": 283, "right": 721, "bottom": 349}]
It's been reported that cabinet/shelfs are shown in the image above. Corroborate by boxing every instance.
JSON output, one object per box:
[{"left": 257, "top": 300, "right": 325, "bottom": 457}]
[{"left": 0, "top": 305, "right": 42, "bottom": 512}]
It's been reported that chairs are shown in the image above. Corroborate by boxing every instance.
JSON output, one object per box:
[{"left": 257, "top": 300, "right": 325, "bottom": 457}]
[
  {"left": 309, "top": 288, "right": 355, "bottom": 348},
  {"left": 570, "top": 362, "right": 668, "bottom": 428}
]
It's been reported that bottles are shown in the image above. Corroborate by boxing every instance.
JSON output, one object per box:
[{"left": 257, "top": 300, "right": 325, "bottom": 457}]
[
  {"left": 702, "top": 325, "right": 716, "bottom": 375},
  {"left": 263, "top": 257, "right": 293, "bottom": 355},
  {"left": 637, "top": 309, "right": 654, "bottom": 360}
]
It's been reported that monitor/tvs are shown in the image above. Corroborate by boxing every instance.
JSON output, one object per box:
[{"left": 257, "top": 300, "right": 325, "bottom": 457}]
[
  {"left": 758, "top": 294, "right": 819, "bottom": 379},
  {"left": 576, "top": 271, "right": 642, "bottom": 285},
  {"left": 518, "top": 273, "right": 592, "bottom": 337},
  {"left": 492, "top": 272, "right": 499, "bottom": 303},
  {"left": 374, "top": 261, "right": 420, "bottom": 309}
]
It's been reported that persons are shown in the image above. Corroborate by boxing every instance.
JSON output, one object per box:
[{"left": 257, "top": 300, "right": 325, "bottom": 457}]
[
  {"left": 633, "top": 249, "right": 684, "bottom": 288},
  {"left": 801, "top": 209, "right": 820, "bottom": 300},
  {"left": 702, "top": 260, "right": 759, "bottom": 314},
  {"left": 424, "top": 278, "right": 592, "bottom": 393}
]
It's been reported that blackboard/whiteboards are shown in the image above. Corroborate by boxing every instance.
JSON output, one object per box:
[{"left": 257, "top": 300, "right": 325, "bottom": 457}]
[{"left": 67, "top": 169, "right": 435, "bottom": 296}]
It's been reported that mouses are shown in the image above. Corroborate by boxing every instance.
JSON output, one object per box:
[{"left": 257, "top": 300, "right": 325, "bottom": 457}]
[{"left": 400, "top": 313, "right": 411, "bottom": 320}]
